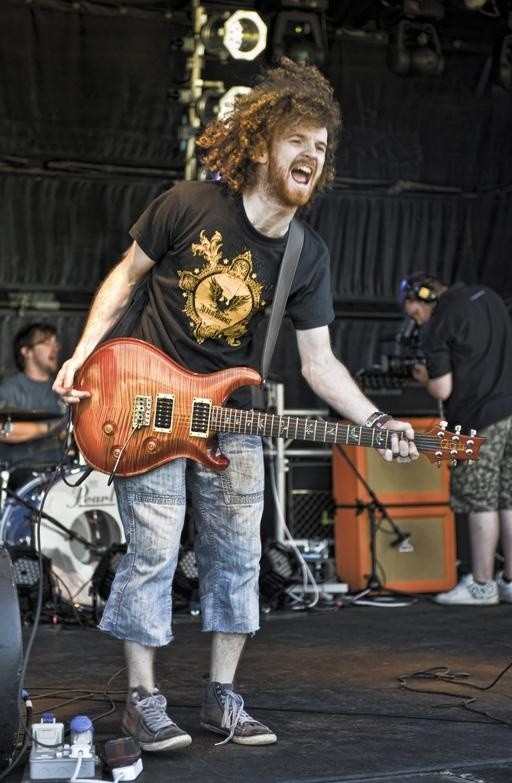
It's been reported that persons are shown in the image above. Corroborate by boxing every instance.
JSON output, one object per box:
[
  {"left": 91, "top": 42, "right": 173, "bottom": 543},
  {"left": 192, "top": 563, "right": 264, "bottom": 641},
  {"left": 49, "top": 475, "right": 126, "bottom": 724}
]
[
  {"left": 1, "top": 320, "right": 82, "bottom": 555},
  {"left": 401, "top": 269, "right": 511, "bottom": 606},
  {"left": 52, "top": 57, "right": 421, "bottom": 753}
]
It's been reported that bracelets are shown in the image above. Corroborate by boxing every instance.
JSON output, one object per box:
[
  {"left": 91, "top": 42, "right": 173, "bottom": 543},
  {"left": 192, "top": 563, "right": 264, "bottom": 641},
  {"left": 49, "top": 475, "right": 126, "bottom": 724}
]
[{"left": 364, "top": 411, "right": 392, "bottom": 428}]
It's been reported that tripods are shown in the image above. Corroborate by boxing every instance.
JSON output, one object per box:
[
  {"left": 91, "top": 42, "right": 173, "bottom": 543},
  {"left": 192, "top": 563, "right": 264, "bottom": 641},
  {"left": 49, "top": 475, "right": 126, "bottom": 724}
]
[{"left": 314, "top": 411, "right": 418, "bottom": 604}]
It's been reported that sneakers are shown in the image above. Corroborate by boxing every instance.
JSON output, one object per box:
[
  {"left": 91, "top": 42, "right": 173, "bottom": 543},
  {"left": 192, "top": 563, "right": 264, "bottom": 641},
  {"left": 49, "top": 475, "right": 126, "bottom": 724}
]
[
  {"left": 120, "top": 684, "right": 191, "bottom": 754},
  {"left": 430, "top": 568, "right": 512, "bottom": 606},
  {"left": 197, "top": 678, "right": 280, "bottom": 750}
]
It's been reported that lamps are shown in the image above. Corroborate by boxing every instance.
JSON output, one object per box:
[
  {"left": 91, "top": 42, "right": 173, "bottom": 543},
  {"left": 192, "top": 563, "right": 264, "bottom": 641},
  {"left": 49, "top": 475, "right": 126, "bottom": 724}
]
[
  {"left": 263, "top": 6, "right": 322, "bottom": 67},
  {"left": 387, "top": 15, "right": 448, "bottom": 80},
  {"left": 172, "top": 0, "right": 268, "bottom": 180},
  {"left": 175, "top": 548, "right": 201, "bottom": 614},
  {"left": 90, "top": 540, "right": 128, "bottom": 628},
  {"left": 259, "top": 543, "right": 302, "bottom": 612},
  {"left": 9, "top": 542, "right": 59, "bottom": 624}
]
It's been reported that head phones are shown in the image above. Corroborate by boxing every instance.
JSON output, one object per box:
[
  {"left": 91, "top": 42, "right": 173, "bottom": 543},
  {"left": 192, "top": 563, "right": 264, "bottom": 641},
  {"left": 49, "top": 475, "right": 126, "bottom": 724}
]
[{"left": 401, "top": 279, "right": 439, "bottom": 303}]
